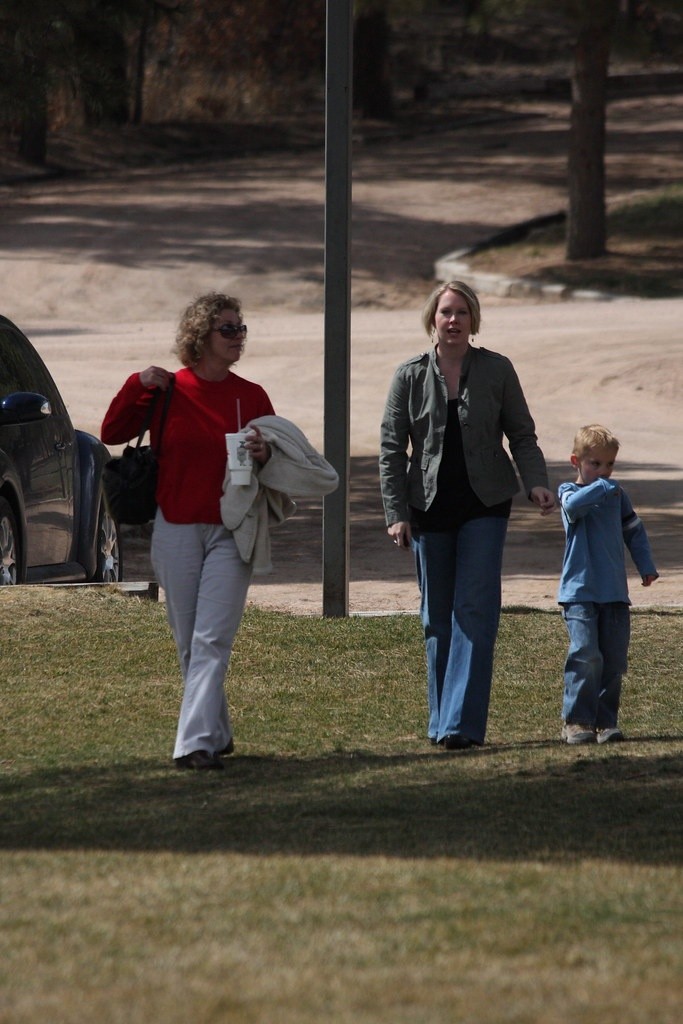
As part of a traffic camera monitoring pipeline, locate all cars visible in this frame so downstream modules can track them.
[0,311,125,588]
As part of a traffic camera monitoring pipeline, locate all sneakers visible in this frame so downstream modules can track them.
[596,725,624,744]
[561,722,598,745]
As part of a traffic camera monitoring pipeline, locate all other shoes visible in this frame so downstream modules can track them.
[175,750,225,771]
[219,738,234,755]
[430,739,442,745]
[444,734,474,749]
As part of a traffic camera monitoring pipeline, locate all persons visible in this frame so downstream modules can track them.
[558,424,660,746]
[378,280,557,750]
[100,290,276,773]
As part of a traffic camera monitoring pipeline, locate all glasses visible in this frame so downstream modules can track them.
[212,323,247,340]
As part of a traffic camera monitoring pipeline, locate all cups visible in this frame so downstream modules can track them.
[224,433,253,485]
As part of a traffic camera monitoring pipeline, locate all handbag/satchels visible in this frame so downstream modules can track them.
[102,371,175,526]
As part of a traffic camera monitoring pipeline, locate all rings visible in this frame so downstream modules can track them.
[259,442,264,450]
[394,539,397,544]
